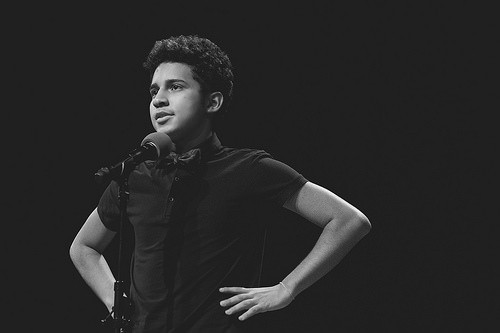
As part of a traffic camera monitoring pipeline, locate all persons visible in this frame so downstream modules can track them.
[69,35,372,333]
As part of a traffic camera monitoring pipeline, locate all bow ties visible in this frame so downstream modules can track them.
[155,148,203,174]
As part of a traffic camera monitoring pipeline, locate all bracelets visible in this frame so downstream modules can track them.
[280,281,295,300]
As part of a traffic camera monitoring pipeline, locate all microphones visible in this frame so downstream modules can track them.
[94,132,173,184]
